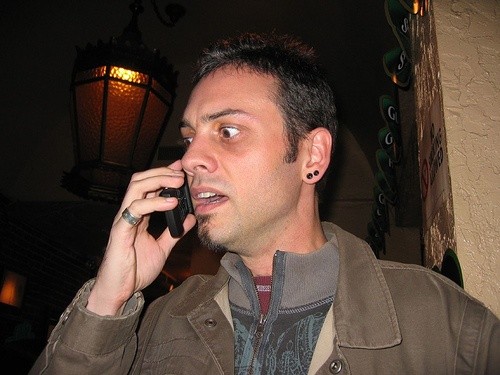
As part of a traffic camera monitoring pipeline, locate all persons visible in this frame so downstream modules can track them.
[31,31,500,374]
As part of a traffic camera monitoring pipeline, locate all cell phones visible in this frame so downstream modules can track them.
[159,167,193,238]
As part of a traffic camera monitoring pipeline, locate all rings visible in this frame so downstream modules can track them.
[120,209,141,228]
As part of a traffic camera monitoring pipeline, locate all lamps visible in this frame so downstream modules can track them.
[61,6,180,206]
